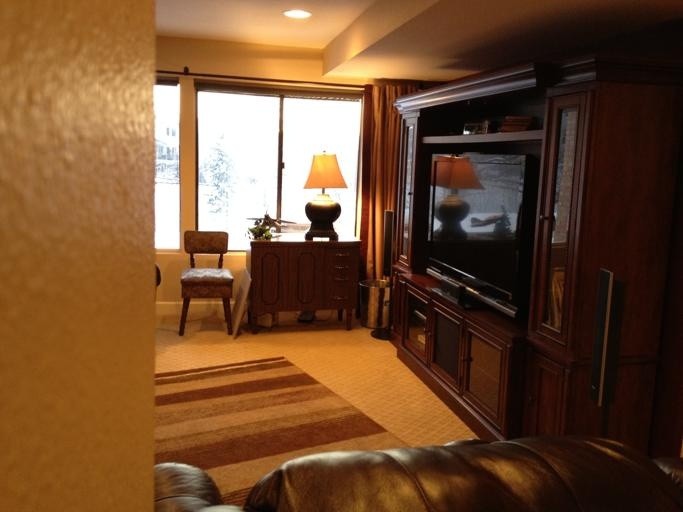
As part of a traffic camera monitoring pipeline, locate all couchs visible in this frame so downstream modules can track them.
[154,434,683,512]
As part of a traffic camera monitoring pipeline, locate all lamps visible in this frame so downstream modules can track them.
[304,151,347,241]
[431,154,485,242]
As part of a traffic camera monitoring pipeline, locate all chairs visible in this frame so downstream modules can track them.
[179,231,234,336]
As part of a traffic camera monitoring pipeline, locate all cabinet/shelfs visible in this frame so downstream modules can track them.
[522,348,657,459]
[391,271,528,442]
[524,81,680,362]
[245,232,362,331]
[394,110,461,270]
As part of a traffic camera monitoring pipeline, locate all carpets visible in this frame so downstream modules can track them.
[155,355,413,510]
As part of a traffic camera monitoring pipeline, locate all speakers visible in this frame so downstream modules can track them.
[589,267,614,408]
[383,210,394,278]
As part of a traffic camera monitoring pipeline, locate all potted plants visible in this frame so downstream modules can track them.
[248,219,272,240]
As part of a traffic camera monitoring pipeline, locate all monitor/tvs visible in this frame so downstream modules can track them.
[424,155,540,317]
